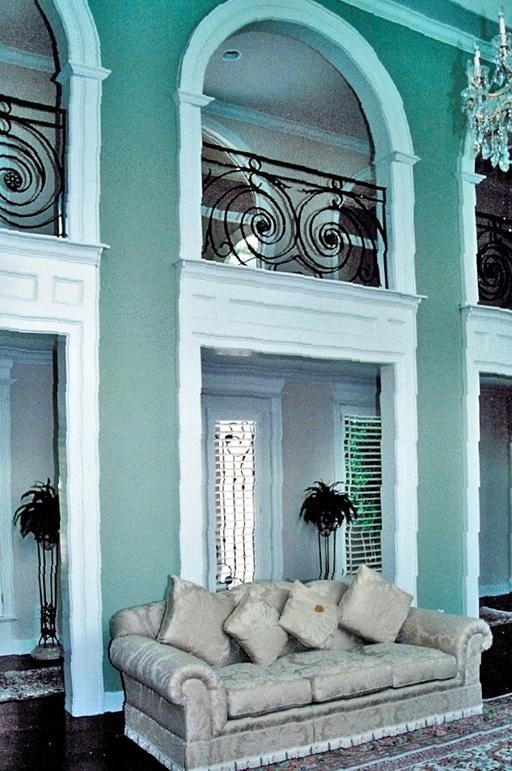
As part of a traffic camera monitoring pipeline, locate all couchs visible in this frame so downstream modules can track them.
[108,581,496,770]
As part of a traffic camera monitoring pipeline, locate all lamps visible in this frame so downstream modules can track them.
[460,5,511,173]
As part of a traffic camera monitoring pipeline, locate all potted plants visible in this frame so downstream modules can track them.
[14,478,61,661]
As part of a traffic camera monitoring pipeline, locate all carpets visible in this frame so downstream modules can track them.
[0,666,65,703]
[247,691,512,770]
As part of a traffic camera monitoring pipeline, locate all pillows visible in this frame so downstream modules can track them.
[338,565,415,644]
[280,580,343,652]
[157,574,234,667]
[221,586,289,665]
